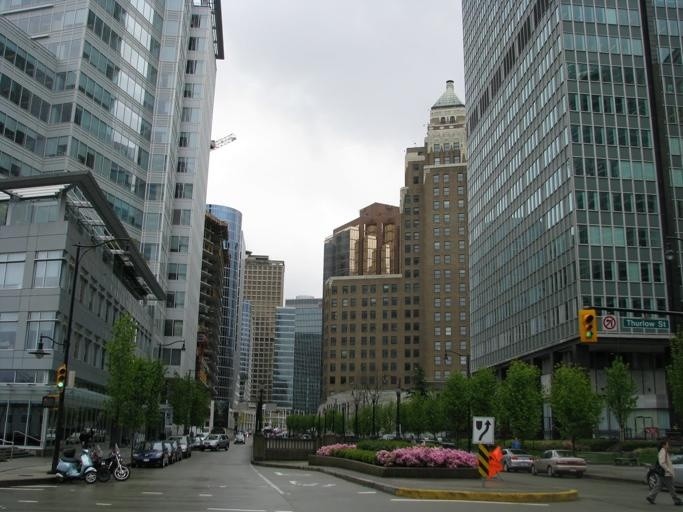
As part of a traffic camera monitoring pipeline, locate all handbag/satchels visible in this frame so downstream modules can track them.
[653,460,666,476]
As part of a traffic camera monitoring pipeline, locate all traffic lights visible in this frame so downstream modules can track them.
[56,366,66,391]
[578,307,598,344]
[42,396,55,409]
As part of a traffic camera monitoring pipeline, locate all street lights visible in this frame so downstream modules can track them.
[442,349,472,451]
[157,340,186,359]
[255,387,266,432]
[370,394,378,439]
[393,386,403,441]
[354,400,361,440]
[24,237,133,477]
[318,403,347,440]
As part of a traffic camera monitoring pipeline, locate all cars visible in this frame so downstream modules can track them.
[501,448,535,474]
[46,423,106,447]
[646,454,682,494]
[132,430,252,469]
[530,449,587,481]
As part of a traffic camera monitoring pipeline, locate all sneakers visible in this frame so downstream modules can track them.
[674,501,683,506]
[646,496,657,504]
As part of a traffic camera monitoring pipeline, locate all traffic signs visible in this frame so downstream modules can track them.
[623,317,669,329]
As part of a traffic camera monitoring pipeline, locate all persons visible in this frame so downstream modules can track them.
[512,437,522,449]
[79,429,88,448]
[645,440,683,505]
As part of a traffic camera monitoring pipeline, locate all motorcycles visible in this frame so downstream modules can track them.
[53,443,130,485]
